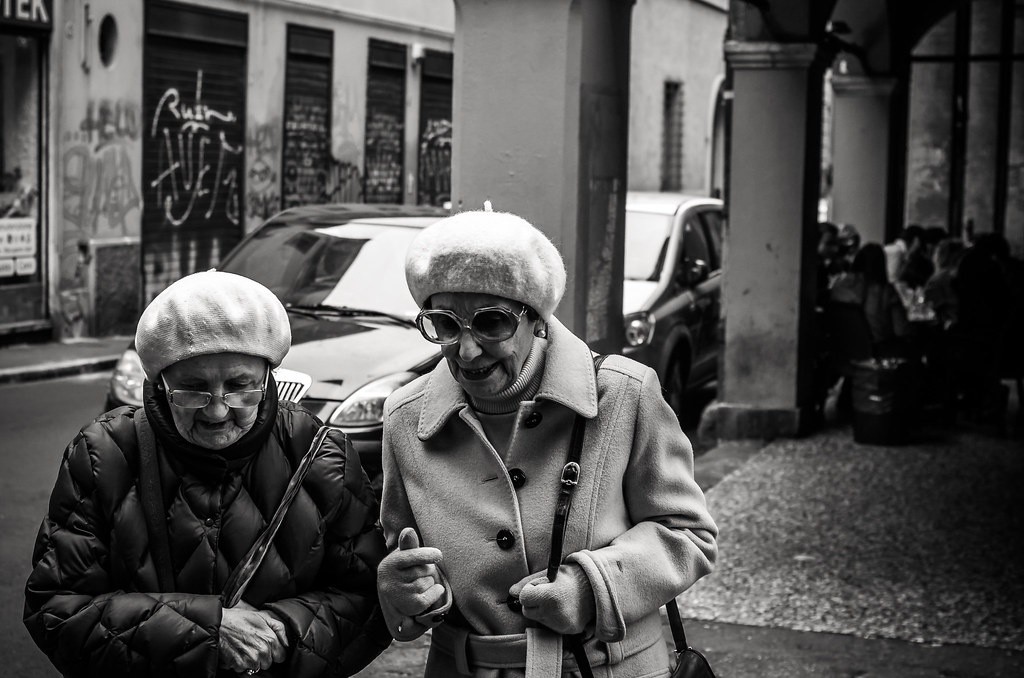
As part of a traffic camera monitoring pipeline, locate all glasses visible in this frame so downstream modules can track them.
[160,365,270,409]
[415,303,528,345]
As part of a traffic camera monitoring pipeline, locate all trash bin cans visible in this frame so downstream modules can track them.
[849,355,919,448]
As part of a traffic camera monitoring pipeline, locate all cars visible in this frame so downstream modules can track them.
[622,191,726,428]
[100,199,453,515]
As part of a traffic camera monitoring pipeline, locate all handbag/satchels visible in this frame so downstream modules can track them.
[667,647,716,678]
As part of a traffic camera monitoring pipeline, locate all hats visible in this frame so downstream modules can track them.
[134,268,292,381]
[404,201,566,324]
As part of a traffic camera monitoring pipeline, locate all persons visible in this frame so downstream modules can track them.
[377,201,720,678]
[23,268,394,678]
[813,223,1024,423]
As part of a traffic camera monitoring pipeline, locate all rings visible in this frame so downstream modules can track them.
[247,667,260,676]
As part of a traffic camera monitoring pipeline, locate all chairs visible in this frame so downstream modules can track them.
[323,239,394,283]
[820,298,1014,423]
